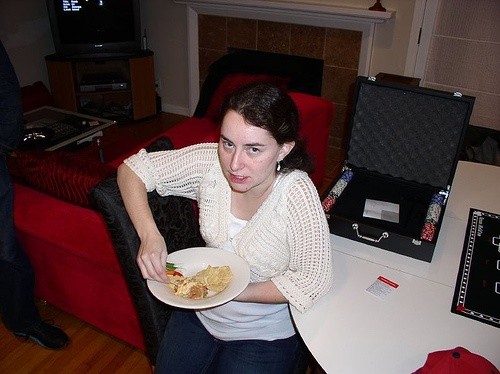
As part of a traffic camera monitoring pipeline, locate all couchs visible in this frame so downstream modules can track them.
[7,74,337,351]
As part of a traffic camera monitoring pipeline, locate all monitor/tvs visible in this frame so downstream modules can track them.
[47,0,141,59]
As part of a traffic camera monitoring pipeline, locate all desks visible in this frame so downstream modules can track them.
[287,161,500,374]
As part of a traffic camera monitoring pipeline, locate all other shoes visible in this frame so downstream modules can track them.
[14,319,71,350]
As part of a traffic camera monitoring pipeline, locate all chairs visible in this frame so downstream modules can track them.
[91,137,310,374]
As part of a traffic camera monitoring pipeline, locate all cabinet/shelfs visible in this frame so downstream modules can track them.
[44,49,159,125]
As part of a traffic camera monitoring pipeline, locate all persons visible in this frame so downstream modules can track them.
[117,81,331,374]
[0,40,71,350]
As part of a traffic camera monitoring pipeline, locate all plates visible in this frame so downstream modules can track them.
[146,246,251,310]
[20,128,56,146]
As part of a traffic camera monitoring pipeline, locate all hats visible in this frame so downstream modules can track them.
[412,346,499,374]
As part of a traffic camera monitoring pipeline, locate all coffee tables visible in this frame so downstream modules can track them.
[20,103,118,164]
